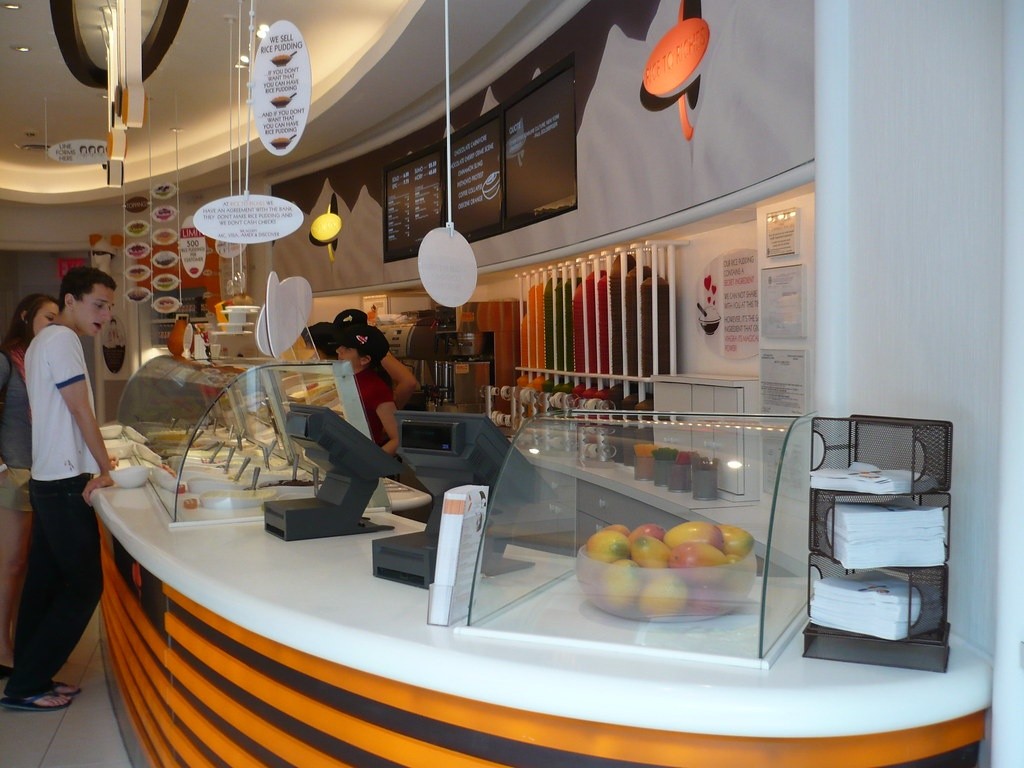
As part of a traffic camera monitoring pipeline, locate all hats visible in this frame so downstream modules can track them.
[333,324,390,361]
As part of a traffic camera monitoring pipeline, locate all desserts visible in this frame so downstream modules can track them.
[128,184,178,310]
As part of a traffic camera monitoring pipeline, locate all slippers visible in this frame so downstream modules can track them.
[50,681,81,695]
[2,693,71,711]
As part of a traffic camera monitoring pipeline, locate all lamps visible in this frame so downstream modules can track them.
[234,20,271,73]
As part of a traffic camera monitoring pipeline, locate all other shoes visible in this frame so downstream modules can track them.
[0,665,14,679]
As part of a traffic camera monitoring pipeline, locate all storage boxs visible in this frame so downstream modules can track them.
[800,413,955,675]
[423,580,472,629]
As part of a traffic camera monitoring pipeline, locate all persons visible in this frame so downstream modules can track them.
[0,266,118,711]
[0,293,61,675]
[301,309,416,479]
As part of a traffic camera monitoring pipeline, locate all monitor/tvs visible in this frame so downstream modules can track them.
[393,408,556,503]
[286,403,406,477]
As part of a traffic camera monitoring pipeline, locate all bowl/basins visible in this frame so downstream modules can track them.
[99,425,322,512]
[269,140,292,149]
[576,545,757,623]
[270,56,292,67]
[270,98,292,109]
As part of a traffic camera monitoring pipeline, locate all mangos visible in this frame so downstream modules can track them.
[587,523,754,614]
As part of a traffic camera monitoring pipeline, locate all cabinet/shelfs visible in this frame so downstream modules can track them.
[649,374,760,502]
[525,456,754,565]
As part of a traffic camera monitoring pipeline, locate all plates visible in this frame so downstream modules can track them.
[125,181,180,313]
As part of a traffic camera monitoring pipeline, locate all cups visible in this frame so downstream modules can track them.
[667,464,692,493]
[691,468,718,500]
[633,455,655,482]
[653,460,674,486]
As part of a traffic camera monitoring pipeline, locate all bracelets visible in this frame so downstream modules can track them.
[0,463,8,473]
